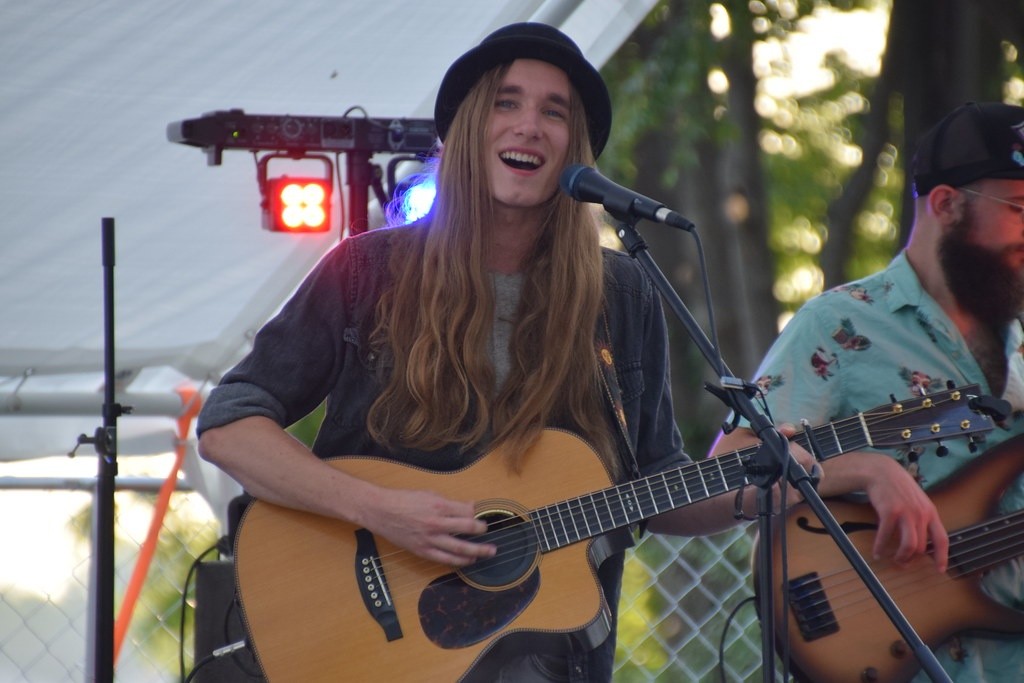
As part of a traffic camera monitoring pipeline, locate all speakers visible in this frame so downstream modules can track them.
[193,560,269,683]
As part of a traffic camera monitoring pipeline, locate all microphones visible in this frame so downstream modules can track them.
[558,162,696,232]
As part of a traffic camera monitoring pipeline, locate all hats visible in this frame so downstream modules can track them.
[435,22,613,164]
[911,102,1024,198]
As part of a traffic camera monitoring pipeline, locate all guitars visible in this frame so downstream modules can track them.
[759,431,1022,683]
[233,375,1011,683]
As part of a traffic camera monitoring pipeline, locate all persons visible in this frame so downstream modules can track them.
[711,95,1024,683]
[198,13,817,683]
